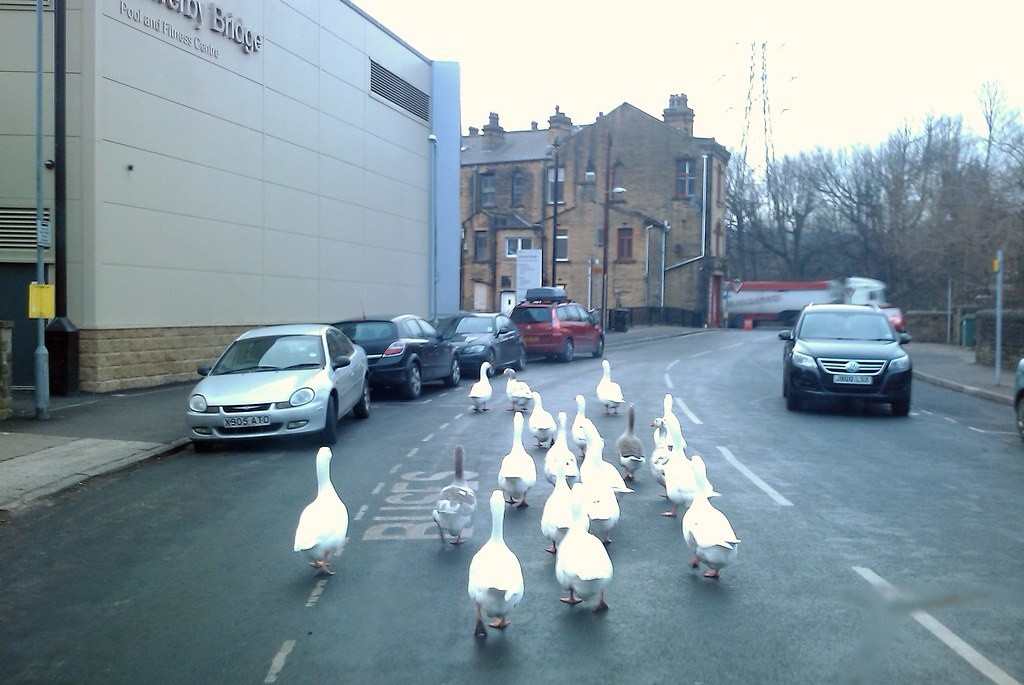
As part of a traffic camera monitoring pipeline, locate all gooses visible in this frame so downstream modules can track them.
[293,445,349,579]
[430,359,742,635]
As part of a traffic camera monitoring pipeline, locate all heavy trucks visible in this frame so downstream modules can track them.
[722,276,888,330]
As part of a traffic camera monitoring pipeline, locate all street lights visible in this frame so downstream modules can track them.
[595,187,627,334]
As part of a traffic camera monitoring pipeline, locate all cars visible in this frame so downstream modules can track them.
[186,323,371,454]
[859,302,911,344]
[778,301,914,417]
[434,311,526,379]
[509,301,605,363]
[1012,357,1024,442]
[330,313,462,401]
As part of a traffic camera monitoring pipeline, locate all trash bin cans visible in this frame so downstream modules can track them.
[615,309,631,331]
